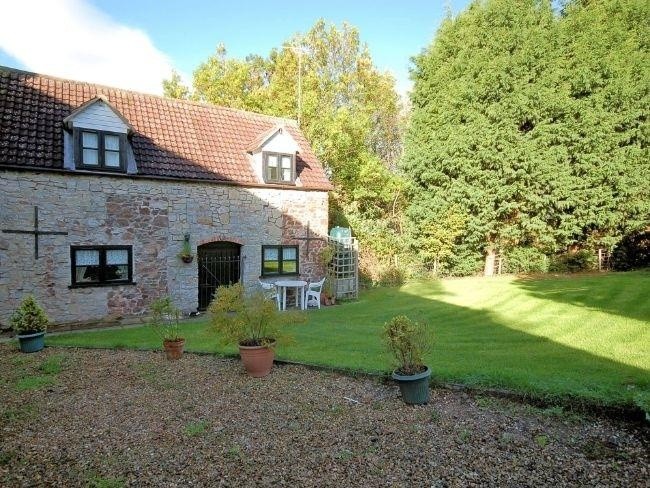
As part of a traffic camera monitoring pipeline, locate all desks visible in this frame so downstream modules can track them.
[275,281,308,311]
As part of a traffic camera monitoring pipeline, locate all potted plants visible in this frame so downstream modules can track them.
[318,245,337,306]
[383,313,434,404]
[181,239,195,264]
[10,296,50,353]
[149,297,186,358]
[209,280,279,377]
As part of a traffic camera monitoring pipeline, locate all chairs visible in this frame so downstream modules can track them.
[304,276,325,310]
